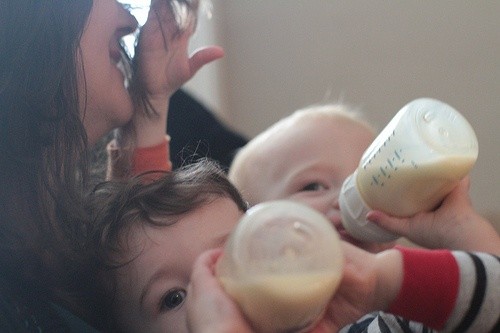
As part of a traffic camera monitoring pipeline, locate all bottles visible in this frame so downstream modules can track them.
[340,99,478,244]
[217,201,342,331]
